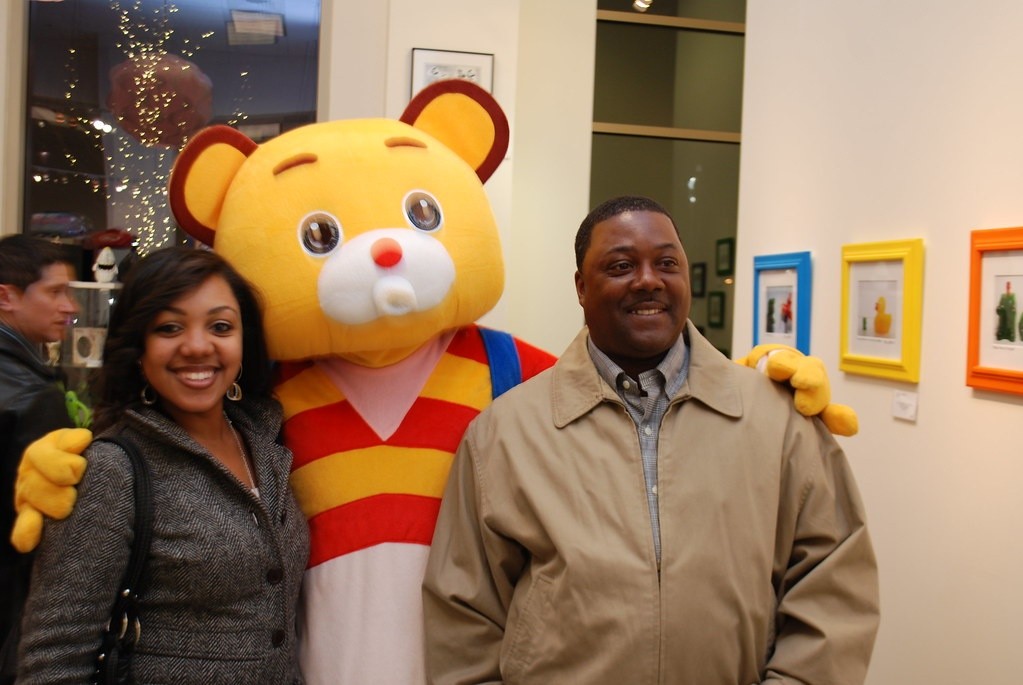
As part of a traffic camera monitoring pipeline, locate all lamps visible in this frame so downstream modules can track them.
[632,0,653,13]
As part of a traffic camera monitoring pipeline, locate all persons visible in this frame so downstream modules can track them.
[13,243,309,685]
[421,193,882,684]
[0,236,83,685]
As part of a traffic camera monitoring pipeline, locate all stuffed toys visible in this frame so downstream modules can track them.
[12,78,861,685]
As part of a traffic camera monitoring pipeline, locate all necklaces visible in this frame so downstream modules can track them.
[226,409,258,497]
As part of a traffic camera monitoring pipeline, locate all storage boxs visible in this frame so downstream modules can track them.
[73,328,108,366]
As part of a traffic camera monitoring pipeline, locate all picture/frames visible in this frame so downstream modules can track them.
[691,263,708,295]
[965,227,1023,397]
[754,251,812,353]
[713,238,735,275]
[838,240,925,383]
[709,291,725,327]
[409,46,495,104]
